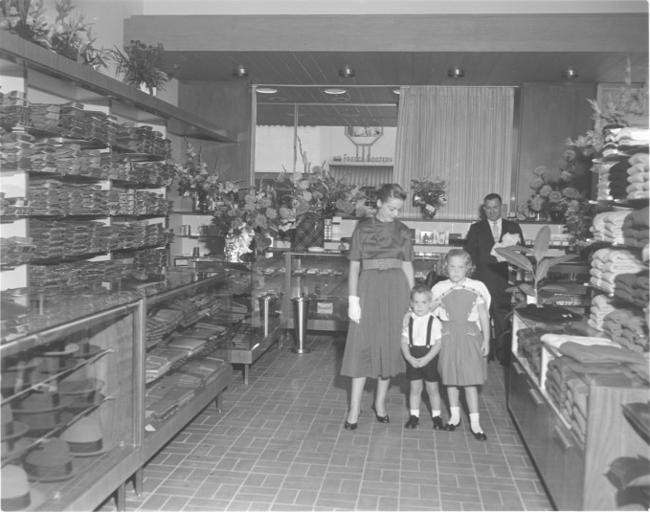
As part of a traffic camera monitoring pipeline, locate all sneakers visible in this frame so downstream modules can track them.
[406,415,419,428]
[432,416,442,430]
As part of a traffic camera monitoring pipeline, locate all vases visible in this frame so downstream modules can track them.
[422,205,436,220]
[292,213,324,253]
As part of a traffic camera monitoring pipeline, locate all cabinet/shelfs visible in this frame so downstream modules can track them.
[505,305,650,512]
[0,29,288,511]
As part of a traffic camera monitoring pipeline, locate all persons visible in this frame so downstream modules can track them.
[430,249,492,440]
[338,182,415,431]
[462,192,527,366]
[400,284,444,430]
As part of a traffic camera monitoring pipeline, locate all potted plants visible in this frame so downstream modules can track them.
[0,0,97,63]
[586,54,649,133]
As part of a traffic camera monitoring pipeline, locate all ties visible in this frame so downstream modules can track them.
[492,222,499,240]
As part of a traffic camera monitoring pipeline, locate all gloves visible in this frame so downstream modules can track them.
[347,295,361,323]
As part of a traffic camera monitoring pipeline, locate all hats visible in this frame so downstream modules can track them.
[1,464,46,512]
[0,332,104,441]
[23,438,76,483]
[59,417,117,457]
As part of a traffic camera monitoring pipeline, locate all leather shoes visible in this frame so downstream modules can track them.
[344,421,357,430]
[444,418,462,431]
[470,425,486,440]
[372,402,389,424]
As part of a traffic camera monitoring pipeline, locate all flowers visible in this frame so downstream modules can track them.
[273,134,372,232]
[515,129,605,242]
[409,175,450,217]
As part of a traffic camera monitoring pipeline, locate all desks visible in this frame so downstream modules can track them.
[282,251,440,355]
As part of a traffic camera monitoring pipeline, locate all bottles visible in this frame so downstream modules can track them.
[180,224,191,237]
[193,246,200,258]
